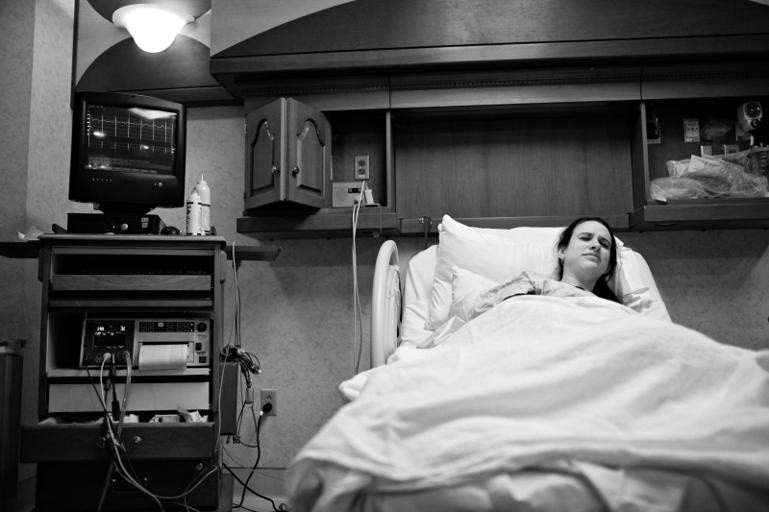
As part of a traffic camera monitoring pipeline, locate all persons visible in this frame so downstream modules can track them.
[555,217,617,292]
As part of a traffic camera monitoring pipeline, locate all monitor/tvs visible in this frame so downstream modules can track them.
[68,91,187,217]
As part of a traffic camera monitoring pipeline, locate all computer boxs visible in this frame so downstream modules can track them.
[65,212,166,236]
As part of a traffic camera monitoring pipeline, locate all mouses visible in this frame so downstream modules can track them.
[160,226,181,236]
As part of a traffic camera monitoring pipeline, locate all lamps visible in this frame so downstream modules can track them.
[88,0,211,53]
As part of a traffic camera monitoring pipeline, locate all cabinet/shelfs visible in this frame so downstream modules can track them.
[33,234,228,512]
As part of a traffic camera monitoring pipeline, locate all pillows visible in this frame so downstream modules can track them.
[424,215,623,328]
[261,389,278,416]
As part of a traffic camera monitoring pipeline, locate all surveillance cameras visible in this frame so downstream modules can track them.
[737,102,763,132]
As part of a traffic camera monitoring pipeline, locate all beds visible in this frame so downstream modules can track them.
[348,215,769,512]
